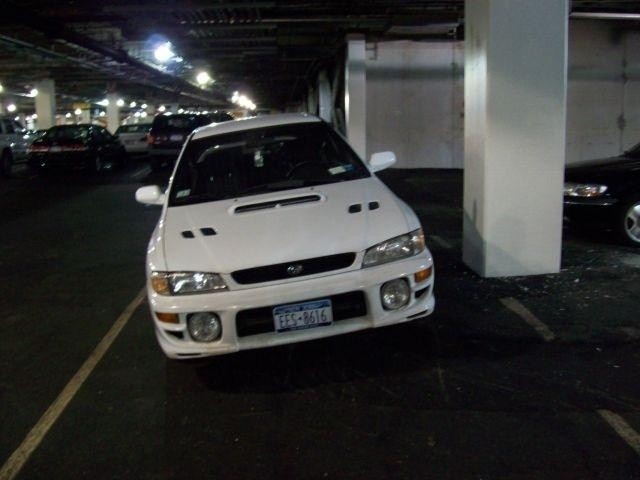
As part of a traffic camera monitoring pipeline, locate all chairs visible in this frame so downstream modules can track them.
[190,156,247,197]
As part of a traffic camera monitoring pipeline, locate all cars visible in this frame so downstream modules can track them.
[564,141,640,251]
[133,114,436,360]
[1,111,235,179]
